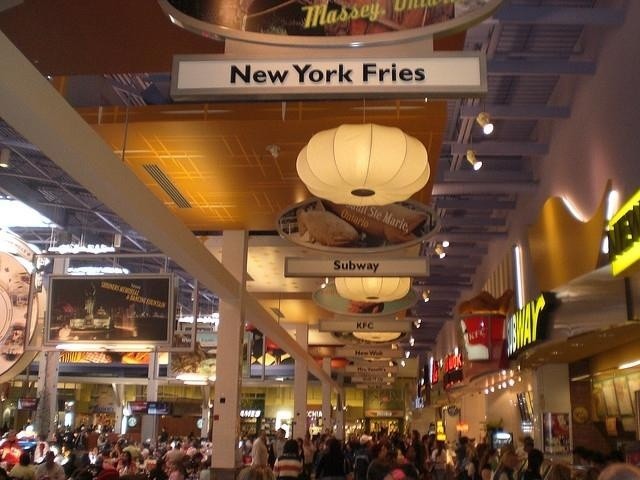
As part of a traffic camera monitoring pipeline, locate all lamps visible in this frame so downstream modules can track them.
[466,150,484,171]
[473,114,494,134]
[297,98,430,206]
[335,277,410,303]
[436,246,446,258]
[423,292,430,303]
